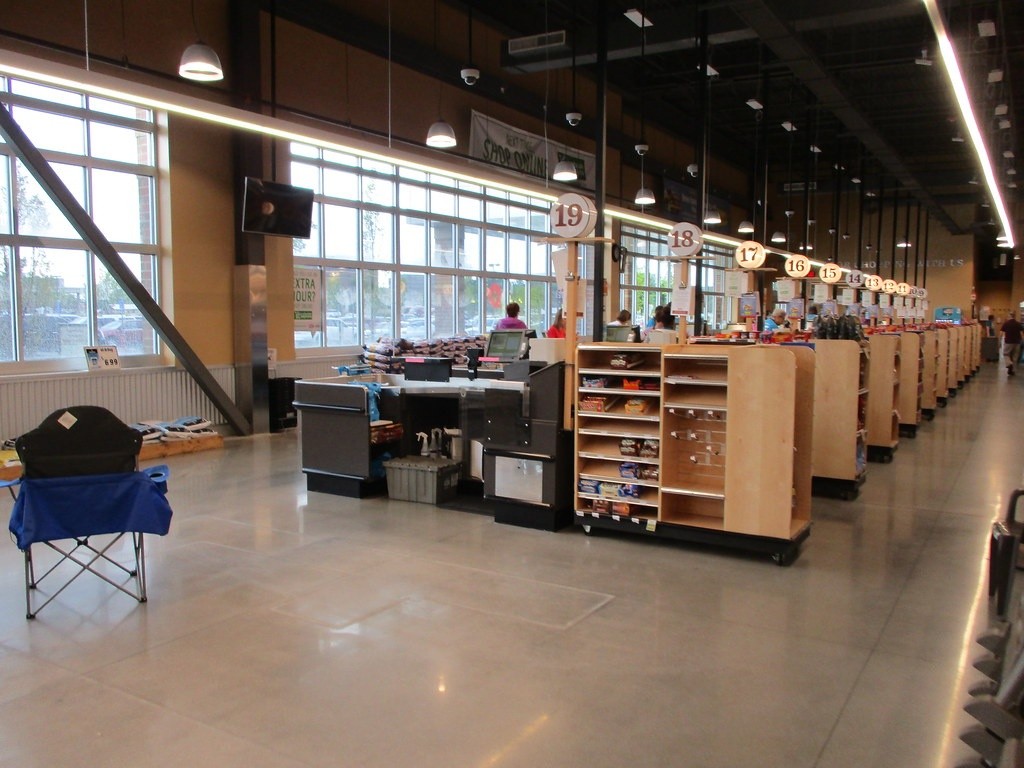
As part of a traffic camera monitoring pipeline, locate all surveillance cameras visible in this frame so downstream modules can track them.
[567,112,582,126]
[635,145,649,155]
[460,69,480,85]
[687,164,698,177]
[785,210,873,250]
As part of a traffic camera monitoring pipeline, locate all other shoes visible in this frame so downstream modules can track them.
[1006,364,1017,376]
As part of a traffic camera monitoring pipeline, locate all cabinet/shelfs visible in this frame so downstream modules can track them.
[573,345,815,561]
[813,322,983,501]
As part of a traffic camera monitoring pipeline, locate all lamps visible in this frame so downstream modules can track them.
[738,217,755,233]
[176,0,226,84]
[551,69,577,186]
[799,241,813,251]
[895,235,913,248]
[996,229,1009,265]
[771,231,787,243]
[425,0,458,148]
[700,208,721,225]
[634,186,656,206]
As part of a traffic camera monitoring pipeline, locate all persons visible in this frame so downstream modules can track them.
[608,310,631,326]
[807,306,818,320]
[644,302,678,343]
[496,303,526,328]
[1000,312,1024,375]
[547,309,578,341]
[764,309,786,331]
[987,315,995,336]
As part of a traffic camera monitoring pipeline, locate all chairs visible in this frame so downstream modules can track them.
[0,406,173,619]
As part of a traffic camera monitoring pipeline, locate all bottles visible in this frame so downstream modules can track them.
[610,354,645,370]
[440,444,449,459]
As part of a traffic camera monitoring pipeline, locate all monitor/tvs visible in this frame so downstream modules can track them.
[606,326,631,342]
[484,329,527,363]
[242,177,314,239]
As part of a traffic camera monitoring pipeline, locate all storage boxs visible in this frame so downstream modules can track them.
[382,458,461,505]
